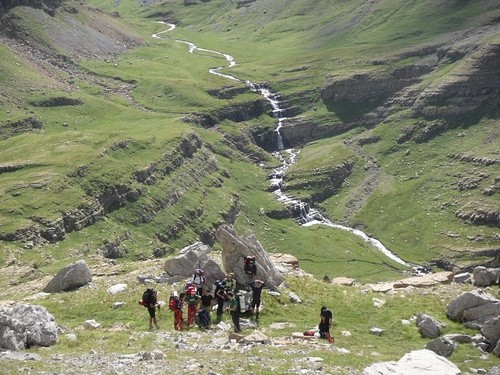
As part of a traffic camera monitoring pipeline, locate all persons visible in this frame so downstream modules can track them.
[244,261,257,286]
[226,287,243,333]
[174,293,185,331]
[148,291,159,330]
[214,275,236,316]
[192,271,203,297]
[319,306,332,339]
[198,289,214,329]
[250,280,265,324]
[185,290,201,326]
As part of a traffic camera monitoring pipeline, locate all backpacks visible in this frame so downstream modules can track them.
[244,256,257,275]
[303,330,315,336]
[169,296,175,311]
[211,280,221,299]
[142,288,153,307]
